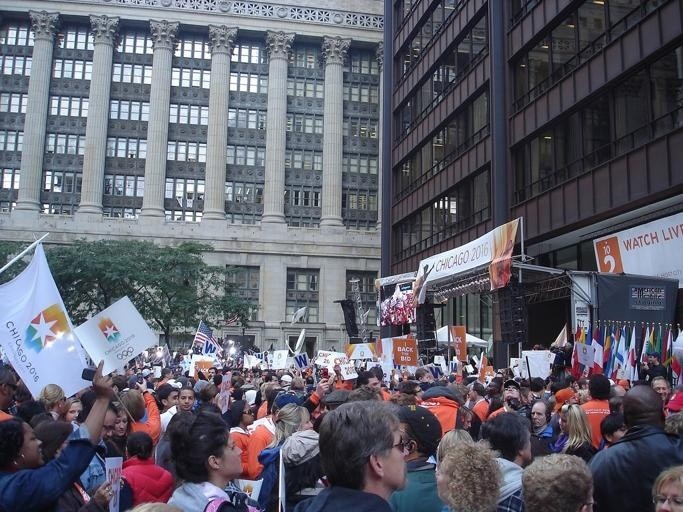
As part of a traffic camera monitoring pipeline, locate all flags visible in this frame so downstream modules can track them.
[71,294,158,377]
[550,326,568,350]
[189,319,222,356]
[372,332,418,365]
[0,244,99,407]
[450,324,467,363]
[224,311,241,328]
[289,306,307,326]
[570,321,681,388]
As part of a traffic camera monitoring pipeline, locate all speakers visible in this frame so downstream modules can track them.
[416,306,436,347]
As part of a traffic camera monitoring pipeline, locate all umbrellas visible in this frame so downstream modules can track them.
[433,324,489,361]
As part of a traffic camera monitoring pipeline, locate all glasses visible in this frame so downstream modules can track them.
[652,495,682,506]
[241,408,253,415]
[505,386,516,391]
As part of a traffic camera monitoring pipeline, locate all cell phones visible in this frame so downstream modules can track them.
[82,368,96,381]
[137,373,143,384]
[320,368,328,383]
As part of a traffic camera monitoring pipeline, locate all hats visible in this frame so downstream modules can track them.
[277,375,291,384]
[323,389,348,404]
[503,380,520,391]
[400,406,442,450]
[141,369,150,377]
[281,430,321,468]
[647,352,659,358]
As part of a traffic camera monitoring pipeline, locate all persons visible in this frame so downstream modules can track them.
[0,341,683,512]
[380,292,415,325]
[495,239,514,287]
[408,264,435,307]
